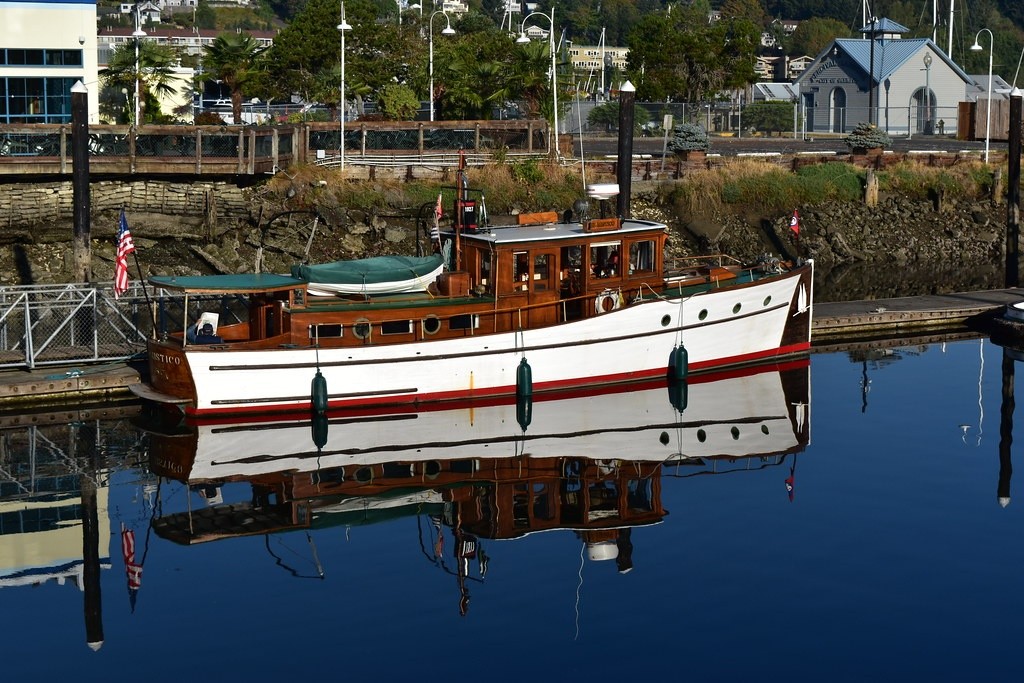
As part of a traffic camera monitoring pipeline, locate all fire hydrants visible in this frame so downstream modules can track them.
[936,119,944,136]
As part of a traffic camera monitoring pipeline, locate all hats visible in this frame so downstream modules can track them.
[202,323,213,336]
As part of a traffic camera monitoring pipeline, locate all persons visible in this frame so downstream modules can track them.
[186,319,225,345]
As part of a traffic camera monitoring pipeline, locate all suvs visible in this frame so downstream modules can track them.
[211,104,272,126]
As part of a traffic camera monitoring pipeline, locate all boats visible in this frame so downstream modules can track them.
[1004,300,1024,320]
[137,356,813,614]
[126,79,815,419]
[291,253,445,296]
[309,488,443,531]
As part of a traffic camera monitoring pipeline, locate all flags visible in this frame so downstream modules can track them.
[433,194,442,221]
[429,513,443,541]
[122,523,143,612]
[434,533,444,556]
[114,207,136,300]
[790,210,799,235]
[785,475,794,502]
[430,210,440,241]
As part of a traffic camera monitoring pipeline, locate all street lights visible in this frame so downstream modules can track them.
[704,104,710,140]
[134,6,148,141]
[398,3,422,31]
[517,13,561,163]
[970,30,993,163]
[429,10,456,122]
[337,0,353,169]
[924,52,932,135]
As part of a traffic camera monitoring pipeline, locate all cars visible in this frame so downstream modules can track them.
[195,99,231,115]
